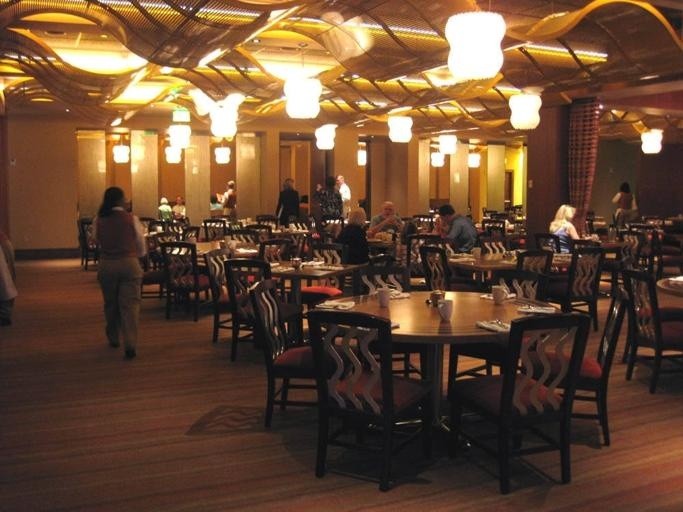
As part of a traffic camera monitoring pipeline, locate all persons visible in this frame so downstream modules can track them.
[159,197,173,223]
[611,182,637,222]
[210,196,222,209]
[92,186,147,358]
[275,175,478,276]
[548,204,594,255]
[173,195,186,220]
[221,180,237,209]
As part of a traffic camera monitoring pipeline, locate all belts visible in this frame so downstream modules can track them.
[101,251,136,259]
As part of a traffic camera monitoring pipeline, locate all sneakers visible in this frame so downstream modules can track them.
[108,339,120,347]
[124,348,136,358]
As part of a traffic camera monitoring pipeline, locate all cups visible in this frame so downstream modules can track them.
[429,288,454,322]
[492,285,509,305]
[376,287,391,308]
[472,247,482,258]
[367,231,375,239]
[218,234,238,251]
[155,226,163,233]
[290,258,302,268]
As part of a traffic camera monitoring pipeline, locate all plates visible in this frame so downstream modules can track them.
[367,239,382,243]
